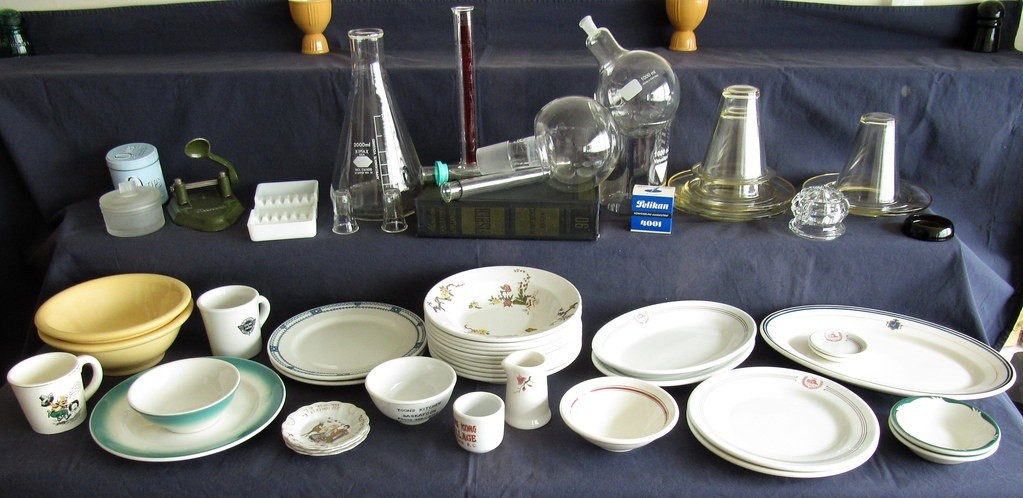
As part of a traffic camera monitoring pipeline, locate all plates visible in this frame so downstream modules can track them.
[282,402,371,457]
[90,357,286,461]
[591,299,757,386]
[423,265,582,384]
[760,303,1016,400]
[687,367,879,478]
[889,396,1002,465]
[267,302,427,386]
[809,331,869,361]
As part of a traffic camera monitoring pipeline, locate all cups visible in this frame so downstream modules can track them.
[332,190,359,235]
[196,285,270,359]
[452,392,504,453]
[381,187,408,232]
[7,351,103,434]
[503,351,552,430]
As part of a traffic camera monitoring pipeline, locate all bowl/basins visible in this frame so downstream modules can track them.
[127,357,241,434]
[364,357,456,425]
[34,273,193,377]
[559,376,679,451]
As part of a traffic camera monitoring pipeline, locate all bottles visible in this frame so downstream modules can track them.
[332,28,425,221]
[579,16,679,133]
[476,96,623,190]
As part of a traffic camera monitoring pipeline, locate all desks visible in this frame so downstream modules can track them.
[0,0,1023,498]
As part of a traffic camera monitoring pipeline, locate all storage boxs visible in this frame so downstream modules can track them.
[630,184,676,234]
[414,176,599,240]
